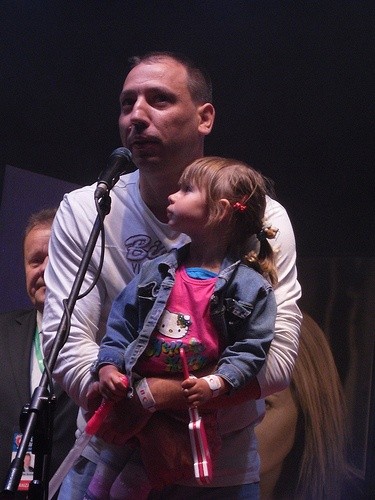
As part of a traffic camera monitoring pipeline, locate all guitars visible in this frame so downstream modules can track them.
[180,347,217,486]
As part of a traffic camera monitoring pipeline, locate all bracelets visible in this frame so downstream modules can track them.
[200,374,222,399]
[134,375,159,415]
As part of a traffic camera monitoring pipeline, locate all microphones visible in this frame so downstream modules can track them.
[93,146,133,199]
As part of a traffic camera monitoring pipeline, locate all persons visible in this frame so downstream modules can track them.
[0,208,81,500]
[41,51,302,500]
[21,453,33,472]
[255,314,364,500]
[85,157,280,500]
